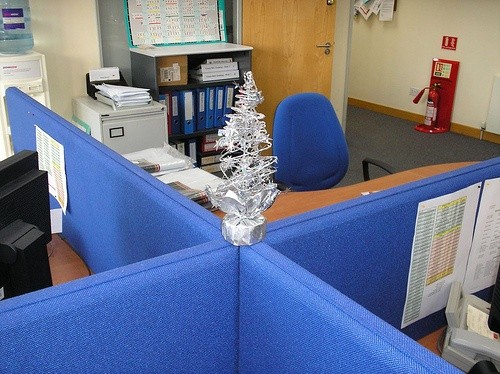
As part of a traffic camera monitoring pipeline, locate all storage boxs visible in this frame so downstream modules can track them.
[156,55,188,87]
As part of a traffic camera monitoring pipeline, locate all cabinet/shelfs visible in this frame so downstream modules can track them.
[129,43,255,177]
[72,94,168,155]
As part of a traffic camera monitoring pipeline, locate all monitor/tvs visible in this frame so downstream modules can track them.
[0,149,54,302]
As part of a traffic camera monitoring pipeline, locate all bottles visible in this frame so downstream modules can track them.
[0,0,34,53]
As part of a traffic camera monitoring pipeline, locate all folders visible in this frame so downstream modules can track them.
[171,85,235,134]
[192,62,240,82]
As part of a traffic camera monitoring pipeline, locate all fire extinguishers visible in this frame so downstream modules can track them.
[423,81,443,129]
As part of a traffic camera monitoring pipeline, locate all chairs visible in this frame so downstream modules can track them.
[271,93,395,191]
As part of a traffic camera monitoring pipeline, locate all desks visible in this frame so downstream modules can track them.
[213,162,478,223]
[46,233,92,286]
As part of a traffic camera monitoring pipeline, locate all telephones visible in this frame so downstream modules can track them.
[441,279,500,373]
